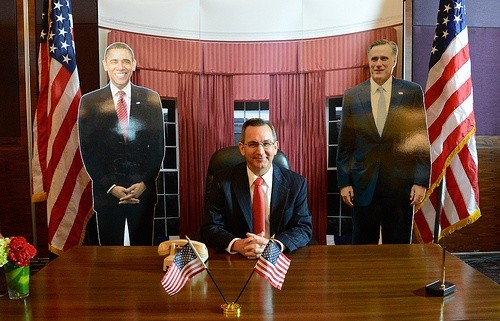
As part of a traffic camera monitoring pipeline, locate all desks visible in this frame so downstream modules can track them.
[0,244,500,321]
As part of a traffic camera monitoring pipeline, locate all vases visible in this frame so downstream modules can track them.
[4,260,30,299]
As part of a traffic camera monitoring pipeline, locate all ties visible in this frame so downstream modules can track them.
[252,177,265,234]
[377,87,388,137]
[116,91,129,142]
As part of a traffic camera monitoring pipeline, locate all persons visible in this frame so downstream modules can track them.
[203,118,313,261]
[336,39,432,246]
[77,42,165,246]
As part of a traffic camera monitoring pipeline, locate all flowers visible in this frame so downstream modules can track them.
[0,235,37,267]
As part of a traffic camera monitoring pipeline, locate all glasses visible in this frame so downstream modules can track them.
[244,142,275,148]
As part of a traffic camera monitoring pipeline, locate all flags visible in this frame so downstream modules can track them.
[160,242,206,296]
[32,1,95,255]
[414,0,482,245]
[254,239,291,290]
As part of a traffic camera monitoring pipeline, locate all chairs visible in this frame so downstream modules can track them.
[199,144,319,245]
